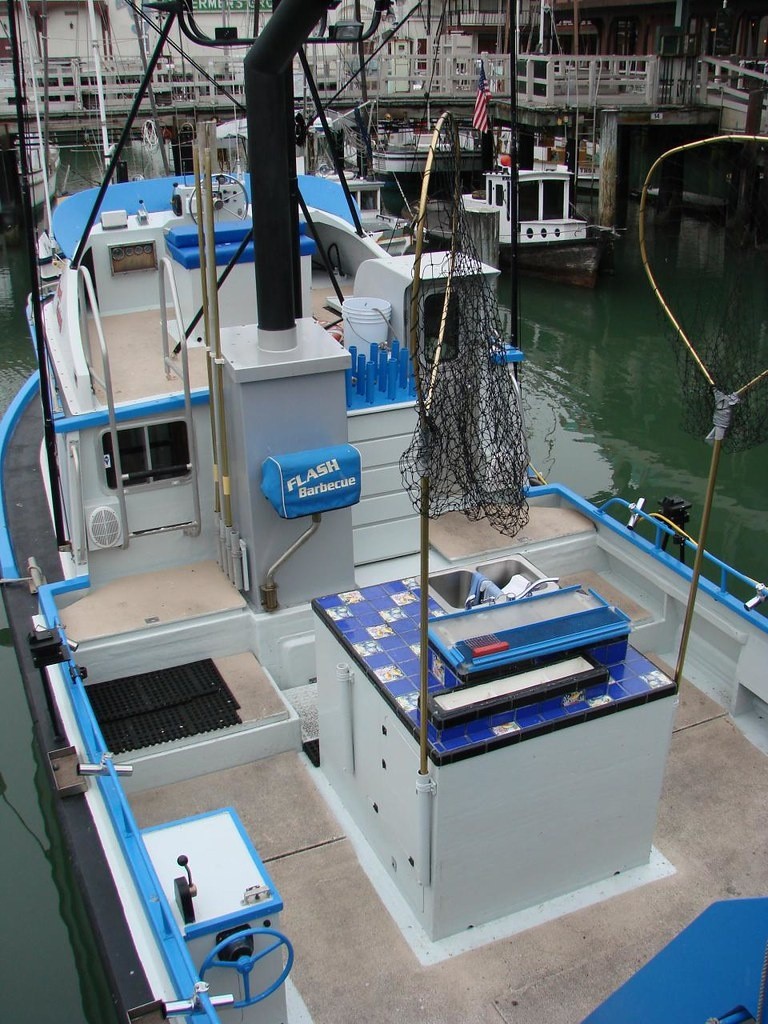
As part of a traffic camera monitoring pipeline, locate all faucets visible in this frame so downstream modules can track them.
[505,577,560,601]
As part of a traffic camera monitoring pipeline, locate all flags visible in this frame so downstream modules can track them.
[471,59,494,135]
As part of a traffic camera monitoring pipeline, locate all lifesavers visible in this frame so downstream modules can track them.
[316,319,344,344]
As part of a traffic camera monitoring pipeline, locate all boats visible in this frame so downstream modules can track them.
[0,0,768,1024]
[461,153,590,245]
[215,106,384,211]
[8,132,61,209]
[342,117,496,176]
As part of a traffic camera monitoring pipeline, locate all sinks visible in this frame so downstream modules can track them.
[476,559,548,597]
[427,566,496,609]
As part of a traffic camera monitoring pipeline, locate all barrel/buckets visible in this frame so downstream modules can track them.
[341,298,393,367]
